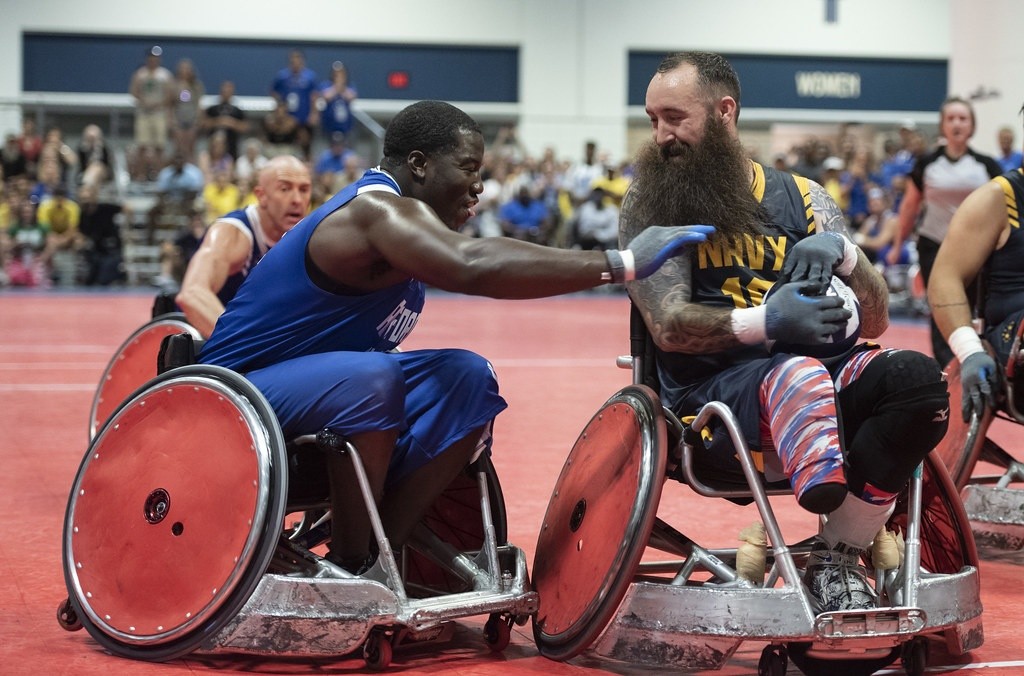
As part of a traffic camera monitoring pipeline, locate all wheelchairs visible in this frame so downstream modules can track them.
[85,291,212,450]
[531,298,987,674]
[930,317,1023,564]
[56,329,542,672]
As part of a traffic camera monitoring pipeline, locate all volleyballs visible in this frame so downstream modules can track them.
[760,270,861,367]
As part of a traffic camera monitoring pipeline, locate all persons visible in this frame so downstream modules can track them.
[616,52,950,616]
[936,156,1024,421]
[190,98,715,579]
[882,97,1007,411]
[173,155,313,343]
[3,34,1024,295]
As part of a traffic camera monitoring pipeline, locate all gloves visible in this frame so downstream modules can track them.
[945,327,1000,418]
[605,223,715,283]
[729,282,851,344]
[781,233,857,292]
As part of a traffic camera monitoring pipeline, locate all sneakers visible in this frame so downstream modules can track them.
[803,537,874,623]
